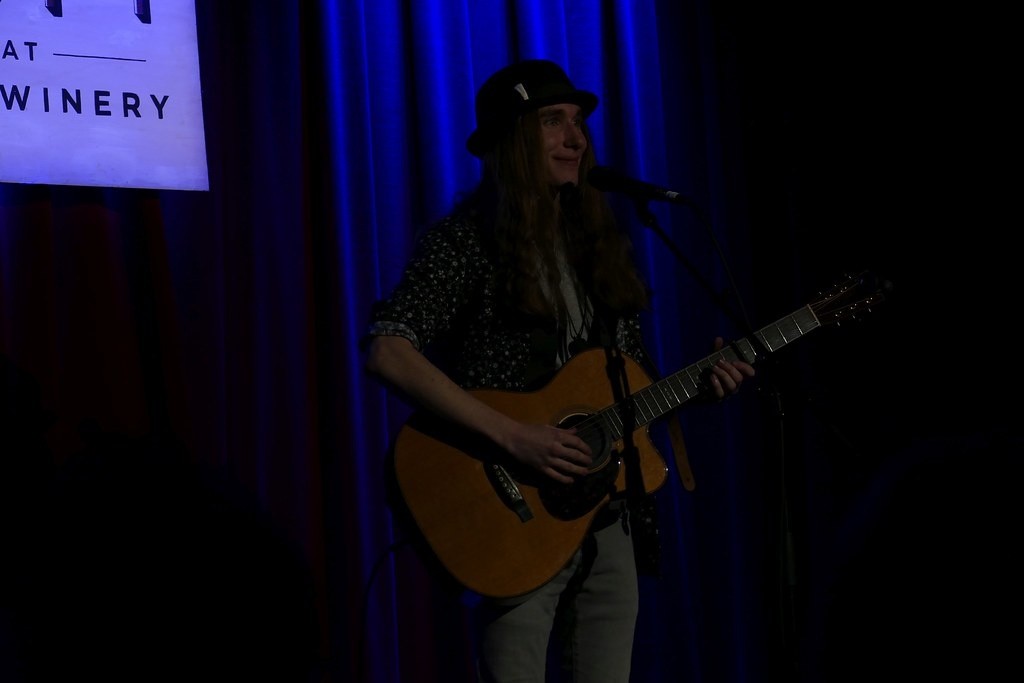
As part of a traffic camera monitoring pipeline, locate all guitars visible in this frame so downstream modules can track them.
[384,261,892,623]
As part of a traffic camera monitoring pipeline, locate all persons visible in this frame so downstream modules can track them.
[359,63,756,682]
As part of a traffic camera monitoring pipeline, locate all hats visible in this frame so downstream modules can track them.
[464,59,600,154]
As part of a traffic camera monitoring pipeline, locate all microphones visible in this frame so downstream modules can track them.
[588,165,680,200]
